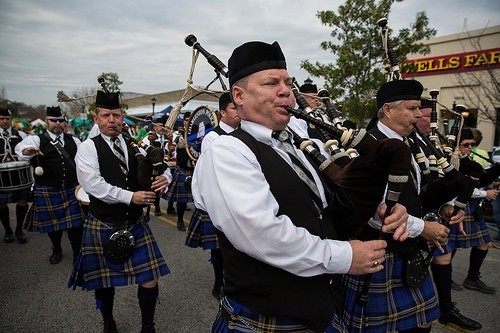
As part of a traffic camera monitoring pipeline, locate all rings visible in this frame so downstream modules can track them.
[373,259,379,267]
[148,199,151,204]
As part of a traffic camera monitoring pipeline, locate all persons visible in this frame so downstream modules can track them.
[0,107,35,245]
[190,42,409,333]
[75,90,173,332]
[0,81,499,333]
[15,105,85,265]
[343,80,467,333]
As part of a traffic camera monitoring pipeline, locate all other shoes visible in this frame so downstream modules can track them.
[49,248,63,265]
[212,286,225,302]
[4,228,27,243]
[177,207,186,231]
[450,276,498,294]
[166,206,176,215]
[438,302,482,331]
[100,319,157,333]
[153,207,162,216]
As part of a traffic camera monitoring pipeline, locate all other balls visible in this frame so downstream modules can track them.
[35,166,43,176]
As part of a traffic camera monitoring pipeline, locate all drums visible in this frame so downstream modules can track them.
[75,184,90,223]
[184,104,223,161]
[0,161,33,192]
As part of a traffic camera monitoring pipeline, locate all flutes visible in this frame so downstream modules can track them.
[376,17,500,268]
[113,90,186,223]
[184,33,412,308]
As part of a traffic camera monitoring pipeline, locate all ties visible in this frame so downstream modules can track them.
[109,136,129,176]
[270,129,323,212]
[2,129,10,139]
[54,136,63,145]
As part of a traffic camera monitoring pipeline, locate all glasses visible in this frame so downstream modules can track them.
[47,118,65,123]
[185,120,189,121]
[460,142,476,148]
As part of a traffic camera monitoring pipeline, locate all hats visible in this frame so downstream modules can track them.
[183,112,192,119]
[96,90,124,110]
[0,108,12,116]
[46,106,64,118]
[376,78,435,110]
[456,128,475,140]
[227,40,288,88]
[153,118,165,124]
[299,83,318,94]
[219,92,232,106]
